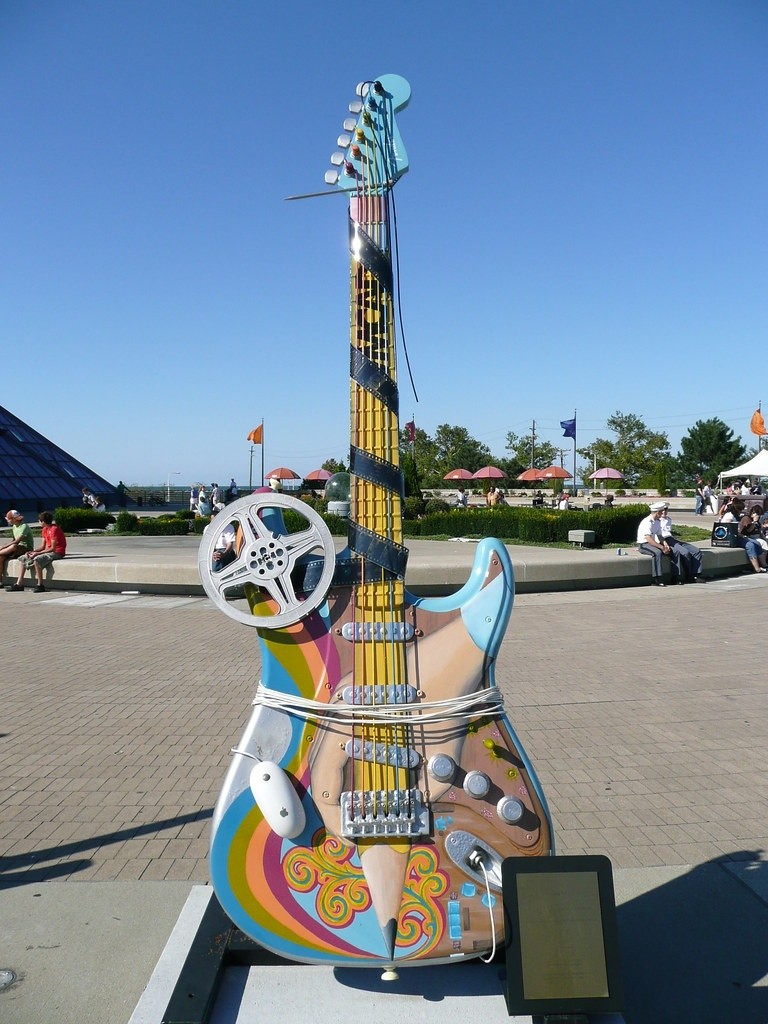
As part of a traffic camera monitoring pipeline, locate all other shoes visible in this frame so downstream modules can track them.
[0,582,4,588]
[651,579,665,586]
[5,584,24,591]
[33,585,45,592]
[755,566,768,573]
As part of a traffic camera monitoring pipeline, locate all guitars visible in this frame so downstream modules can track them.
[199,70,556,983]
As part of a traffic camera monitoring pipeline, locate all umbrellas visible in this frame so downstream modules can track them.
[264,468,301,490]
[516,469,548,499]
[304,470,333,490]
[444,469,477,486]
[471,466,508,486]
[534,466,574,496]
[588,468,625,497]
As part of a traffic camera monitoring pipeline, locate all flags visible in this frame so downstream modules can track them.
[560,408,576,440]
[751,400,768,435]
[247,418,264,444]
[405,412,415,441]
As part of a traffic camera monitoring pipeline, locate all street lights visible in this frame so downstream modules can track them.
[167,470,181,502]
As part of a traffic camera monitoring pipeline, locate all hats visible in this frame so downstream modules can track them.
[663,501,670,508]
[649,502,665,512]
[6,510,24,522]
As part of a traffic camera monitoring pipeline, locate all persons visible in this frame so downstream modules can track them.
[227,478,236,497]
[727,478,762,495]
[190,483,220,517]
[559,494,569,510]
[457,486,467,508]
[695,478,717,517]
[637,501,707,586]
[202,511,236,573]
[0,510,67,592]
[718,496,745,523]
[486,485,503,506]
[738,505,768,573]
[82,487,106,512]
[117,481,131,508]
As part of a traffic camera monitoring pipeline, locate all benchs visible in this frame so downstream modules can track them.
[583,502,622,511]
[518,503,541,509]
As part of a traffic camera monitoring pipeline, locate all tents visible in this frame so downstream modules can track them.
[718,449,768,495]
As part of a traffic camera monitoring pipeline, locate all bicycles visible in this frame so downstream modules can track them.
[149,496,168,507]
